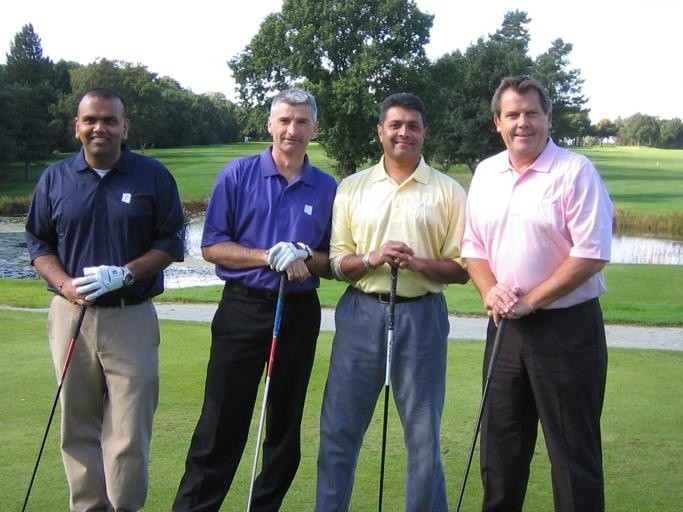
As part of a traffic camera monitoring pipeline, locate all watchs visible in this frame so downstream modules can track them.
[120,265,135,287]
[304,244,312,262]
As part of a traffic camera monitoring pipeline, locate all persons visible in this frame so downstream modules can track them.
[243,134,264,142]
[24,86,186,512]
[312,90,469,512]
[459,75,614,512]
[557,134,617,147]
[140,142,147,154]
[171,88,337,512]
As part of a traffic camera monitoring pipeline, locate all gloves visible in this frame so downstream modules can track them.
[266,241,313,273]
[71,264,124,303]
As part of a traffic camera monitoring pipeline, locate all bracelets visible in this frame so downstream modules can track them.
[361,250,377,272]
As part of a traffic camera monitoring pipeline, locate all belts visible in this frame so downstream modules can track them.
[361,290,432,304]
[91,296,149,307]
[229,284,317,302]
